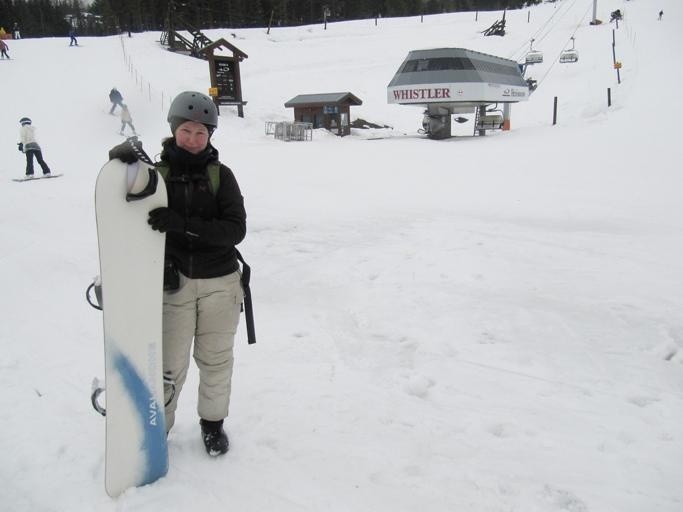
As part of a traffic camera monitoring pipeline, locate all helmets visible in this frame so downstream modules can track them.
[167,91,218,129]
[20,118,31,126]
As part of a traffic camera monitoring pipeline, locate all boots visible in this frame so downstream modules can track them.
[200,418,228,457]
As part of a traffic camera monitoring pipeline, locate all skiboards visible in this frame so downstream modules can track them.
[115,129,142,139]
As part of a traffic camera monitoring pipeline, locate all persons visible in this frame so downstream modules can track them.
[108,92,247,458]
[0,39,10,60]
[658,10,664,20]
[119,104,136,135]
[109,86,123,115]
[68,30,80,46]
[18,117,51,178]
[12,22,22,40]
[609,9,623,29]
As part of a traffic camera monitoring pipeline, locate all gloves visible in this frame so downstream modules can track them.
[148,208,186,234]
[109,136,142,164]
[19,143,23,150]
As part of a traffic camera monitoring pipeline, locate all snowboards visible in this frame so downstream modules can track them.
[11,173,63,182]
[1,58,13,60]
[86,156,168,499]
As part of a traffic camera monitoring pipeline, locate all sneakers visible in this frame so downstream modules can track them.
[27,172,51,178]
